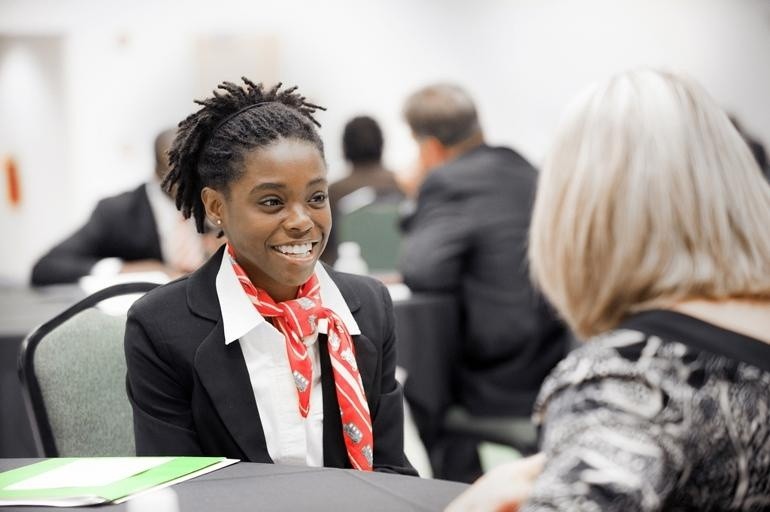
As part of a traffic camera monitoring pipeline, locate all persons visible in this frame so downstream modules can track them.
[458,65,770,512]
[398,82,571,485]
[30,129,227,286]
[125,75,419,476]
[319,116,403,267]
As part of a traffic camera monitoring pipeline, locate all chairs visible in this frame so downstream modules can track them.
[17,280,168,455]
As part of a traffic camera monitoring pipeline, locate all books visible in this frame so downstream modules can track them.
[0,456,240,507]
